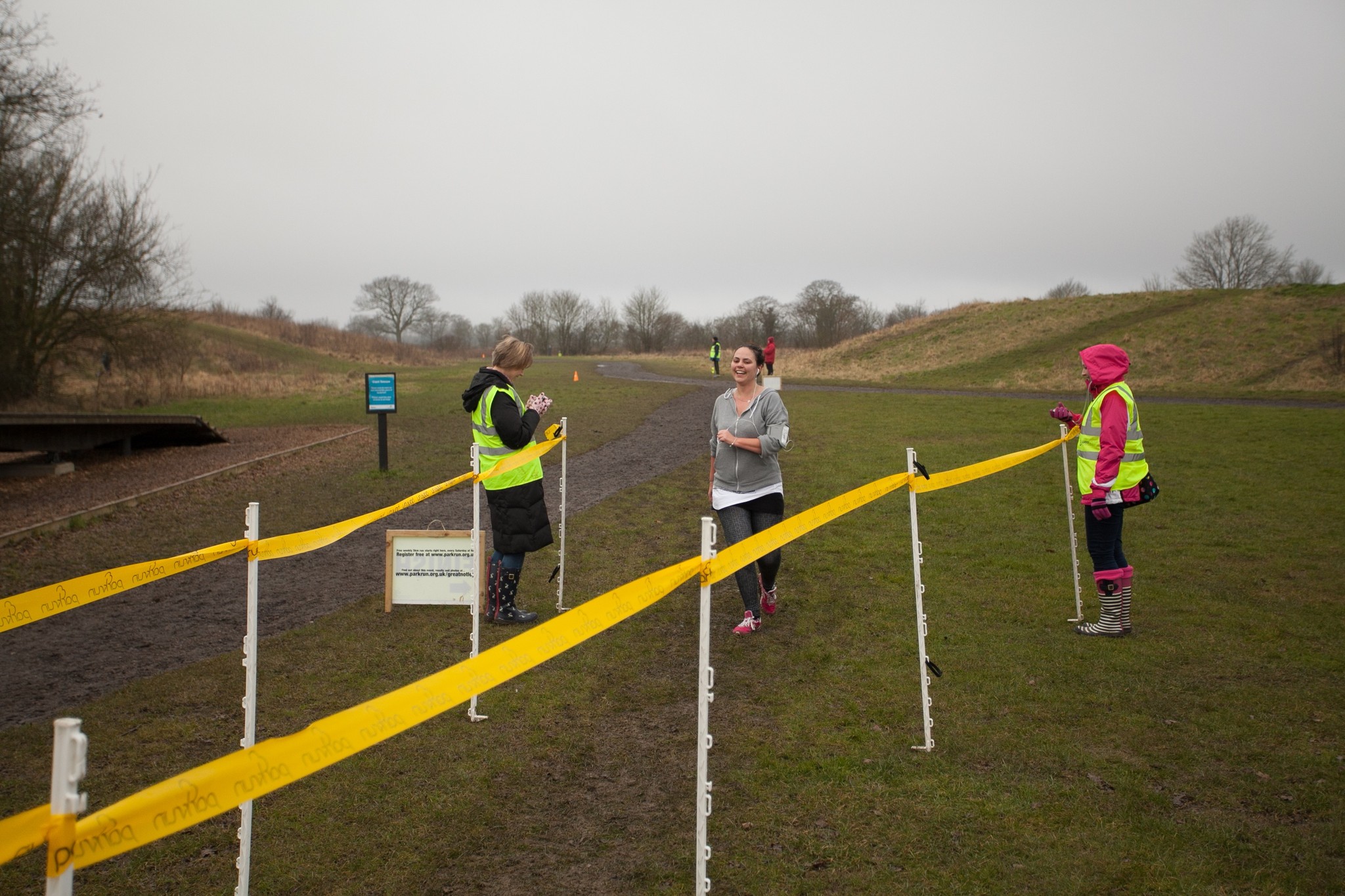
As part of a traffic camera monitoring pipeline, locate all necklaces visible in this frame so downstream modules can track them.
[735,385,758,403]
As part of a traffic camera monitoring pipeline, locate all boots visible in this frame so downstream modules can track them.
[1074,569,1125,638]
[484,556,527,622]
[1119,565,1134,632]
[493,560,538,625]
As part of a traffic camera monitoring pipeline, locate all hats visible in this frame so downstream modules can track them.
[767,337,774,342]
[713,337,718,341]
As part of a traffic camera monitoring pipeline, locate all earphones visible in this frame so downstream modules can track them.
[756,368,760,375]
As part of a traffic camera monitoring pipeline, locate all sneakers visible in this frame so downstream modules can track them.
[731,611,762,636]
[758,573,777,615]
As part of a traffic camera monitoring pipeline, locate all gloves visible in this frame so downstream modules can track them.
[1049,402,1072,422]
[1091,498,1111,520]
[525,392,553,418]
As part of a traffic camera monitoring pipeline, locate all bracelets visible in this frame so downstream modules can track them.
[729,437,738,448]
[709,480,714,484]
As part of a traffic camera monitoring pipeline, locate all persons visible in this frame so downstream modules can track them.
[707,344,789,637]
[710,337,722,378]
[761,337,775,376]
[1049,345,1149,636]
[461,334,553,625]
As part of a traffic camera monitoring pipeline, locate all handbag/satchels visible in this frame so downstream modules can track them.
[1122,471,1160,509]
